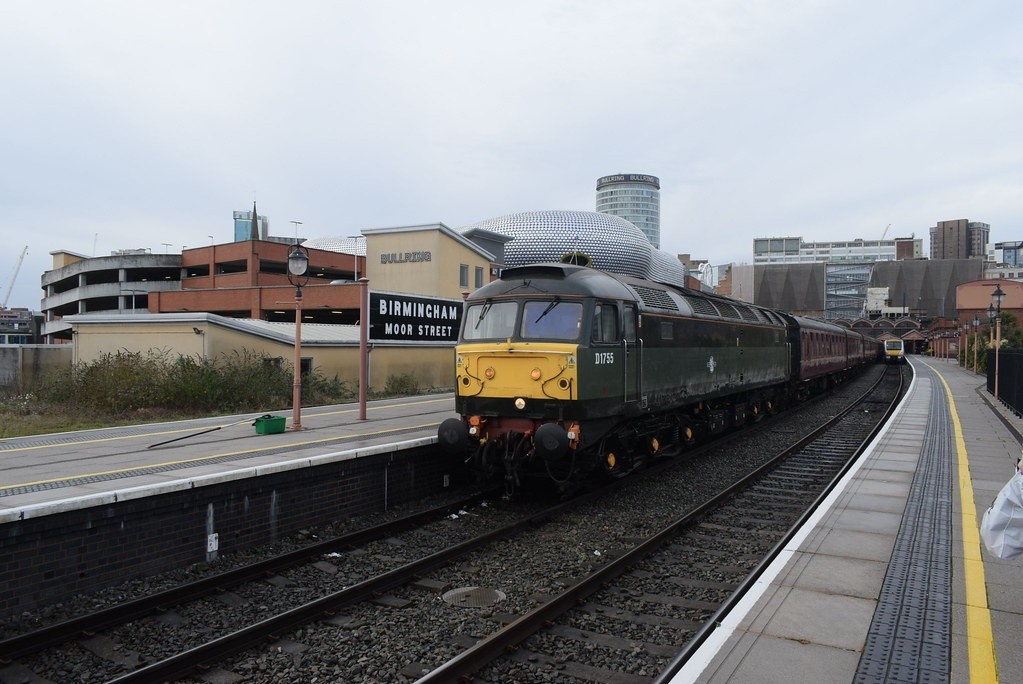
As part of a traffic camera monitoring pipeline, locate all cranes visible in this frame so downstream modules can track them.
[2,245,30,309]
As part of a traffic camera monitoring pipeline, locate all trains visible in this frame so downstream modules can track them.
[438,235,884,503]
[884,339,905,364]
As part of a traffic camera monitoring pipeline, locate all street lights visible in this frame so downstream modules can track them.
[287,239,312,430]
[923,283,1007,406]
